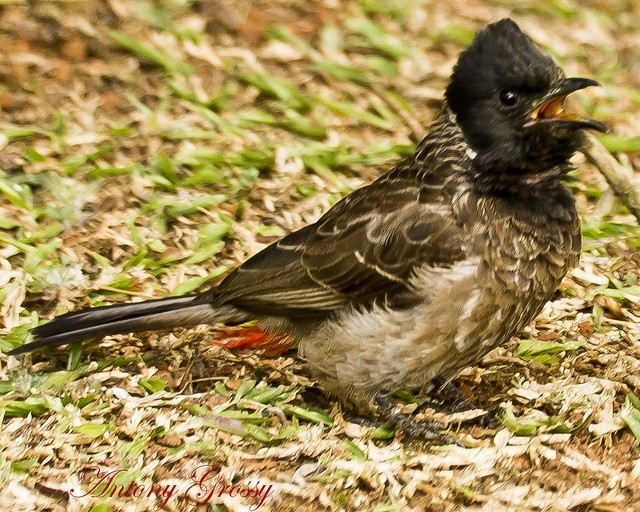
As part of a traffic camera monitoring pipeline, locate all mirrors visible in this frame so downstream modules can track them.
[7,18,611,445]
